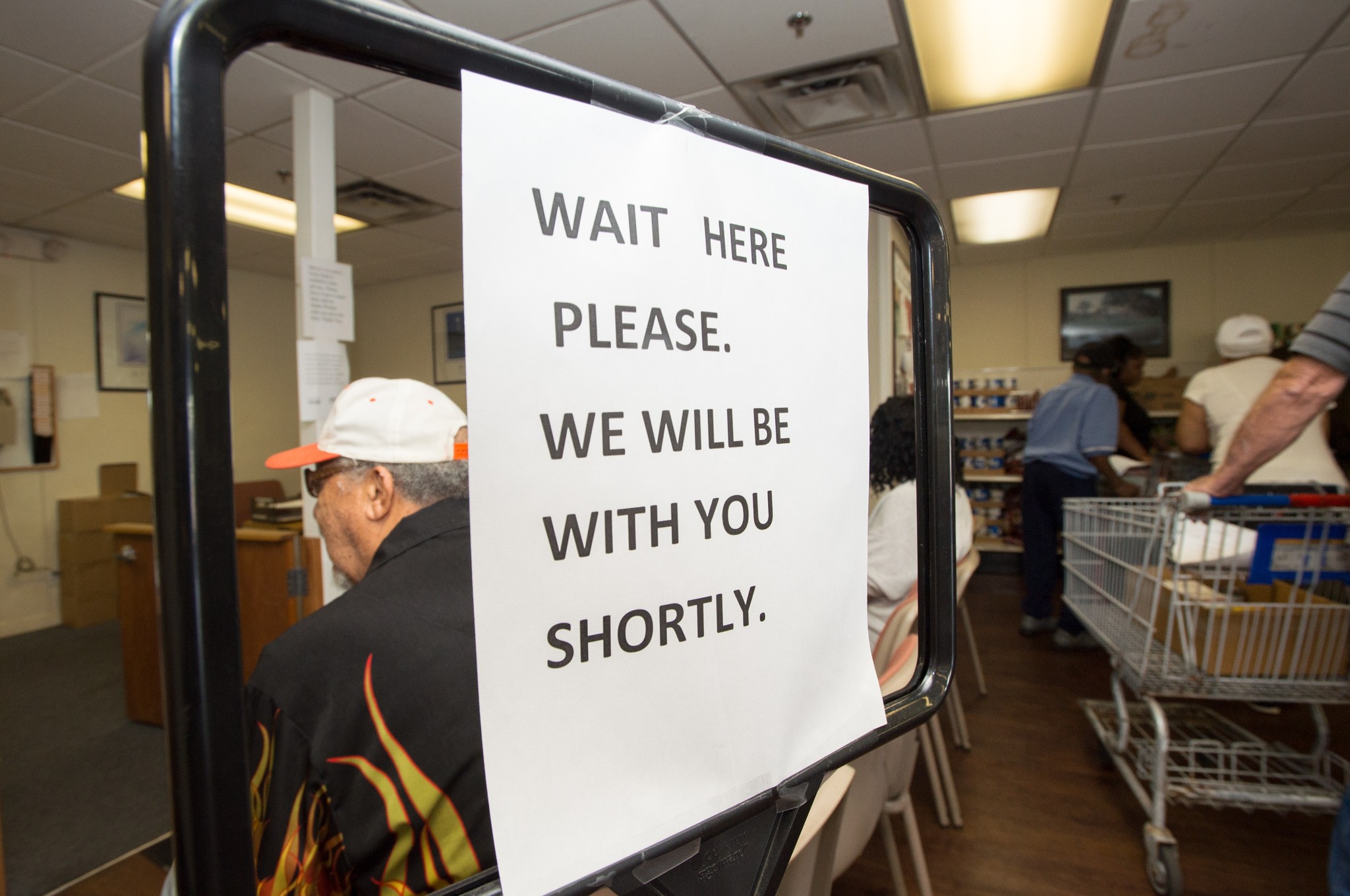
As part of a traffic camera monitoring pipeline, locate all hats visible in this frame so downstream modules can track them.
[1214,314,1274,358]
[264,377,468,469]
[1072,341,1116,370]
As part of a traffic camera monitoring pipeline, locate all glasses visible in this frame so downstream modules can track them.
[304,461,371,498]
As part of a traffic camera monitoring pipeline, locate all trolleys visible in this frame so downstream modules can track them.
[1059,480,1350,896]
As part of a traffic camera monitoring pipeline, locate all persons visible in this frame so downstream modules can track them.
[1021,342,1139,647]
[1177,314,1350,715]
[1098,335,1149,460]
[869,395,971,656]
[1176,267,1350,896]
[159,375,501,896]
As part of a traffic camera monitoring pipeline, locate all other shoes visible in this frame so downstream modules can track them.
[1018,613,1057,634]
[1055,627,1098,647]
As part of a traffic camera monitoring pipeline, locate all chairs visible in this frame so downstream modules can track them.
[786,547,993,896]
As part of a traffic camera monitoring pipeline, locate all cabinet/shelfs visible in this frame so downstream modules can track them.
[950,377,1039,560]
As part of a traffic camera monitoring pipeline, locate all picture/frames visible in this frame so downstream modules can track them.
[1057,277,1174,364]
[889,236,916,397]
[91,288,150,394]
[428,301,468,389]
